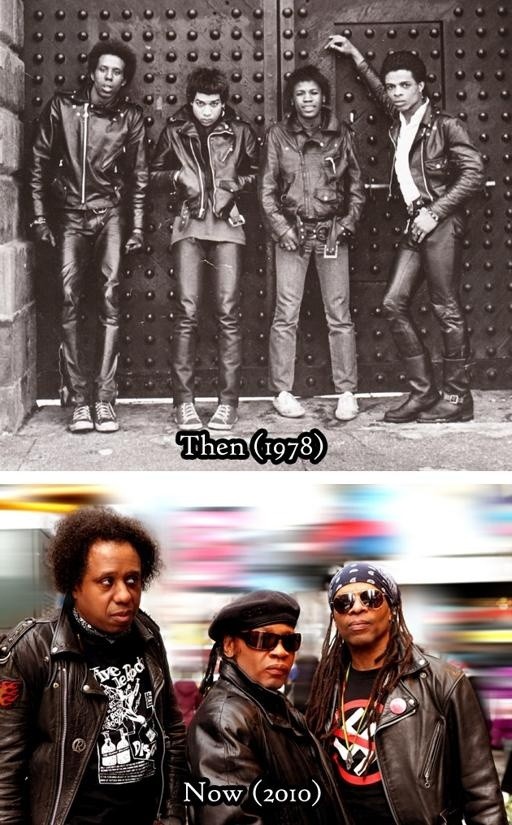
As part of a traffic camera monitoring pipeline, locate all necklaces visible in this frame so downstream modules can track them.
[338,656,394,775]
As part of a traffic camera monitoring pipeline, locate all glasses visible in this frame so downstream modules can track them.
[229,627,303,654]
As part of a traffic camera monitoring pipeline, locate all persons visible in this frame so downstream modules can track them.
[300,559,506,825]
[147,66,259,430]
[1,499,192,823]
[24,39,151,438]
[324,29,488,424]
[180,585,352,824]
[255,62,367,423]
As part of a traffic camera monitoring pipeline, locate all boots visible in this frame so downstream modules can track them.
[383,347,445,423]
[414,354,476,423]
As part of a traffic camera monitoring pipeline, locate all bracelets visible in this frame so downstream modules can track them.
[427,207,440,223]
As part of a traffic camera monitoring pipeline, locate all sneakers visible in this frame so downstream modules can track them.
[207,400,240,431]
[328,588,392,614]
[173,401,204,431]
[68,403,94,432]
[271,389,306,418]
[334,390,360,422]
[92,400,120,433]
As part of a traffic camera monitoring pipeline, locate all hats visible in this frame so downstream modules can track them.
[208,587,301,641]
